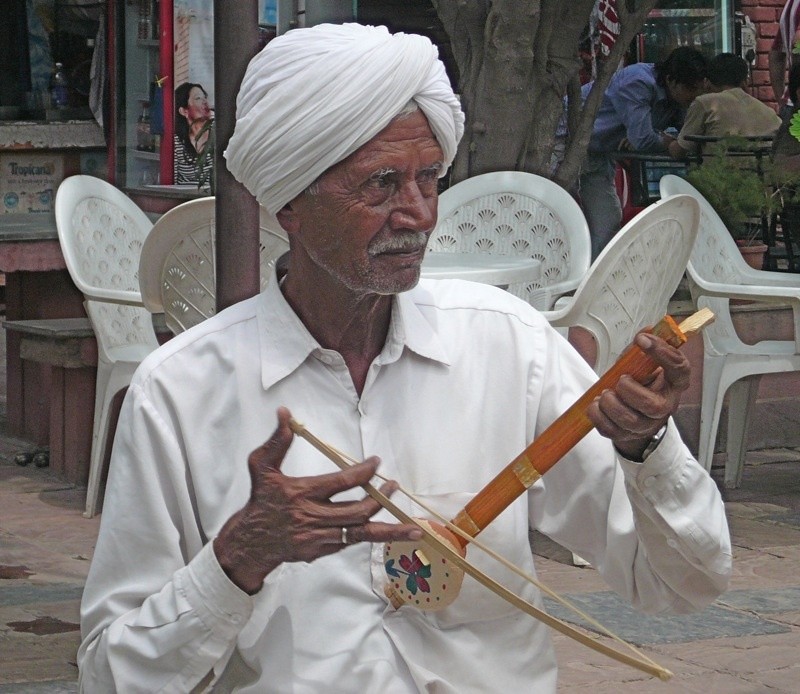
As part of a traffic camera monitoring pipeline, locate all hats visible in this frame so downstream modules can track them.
[225,20,467,220]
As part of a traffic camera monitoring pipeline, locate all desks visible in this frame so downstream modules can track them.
[602,148,698,213]
[418,248,545,294]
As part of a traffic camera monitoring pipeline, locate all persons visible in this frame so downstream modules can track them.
[76,23,733,694]
[550,45,707,268]
[669,52,783,238]
[172,83,213,188]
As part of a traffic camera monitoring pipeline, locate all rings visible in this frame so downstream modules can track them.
[341,525,347,545]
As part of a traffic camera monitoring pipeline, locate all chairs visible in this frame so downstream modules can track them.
[524,190,705,568]
[53,173,161,521]
[682,134,778,241]
[431,162,593,310]
[138,193,289,342]
[658,171,800,492]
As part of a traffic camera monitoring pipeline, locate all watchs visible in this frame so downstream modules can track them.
[640,424,668,462]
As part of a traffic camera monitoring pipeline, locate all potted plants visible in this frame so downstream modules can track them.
[671,131,800,304]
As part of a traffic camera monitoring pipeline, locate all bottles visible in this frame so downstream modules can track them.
[644,24,715,64]
[137,102,152,151]
[50,62,70,111]
